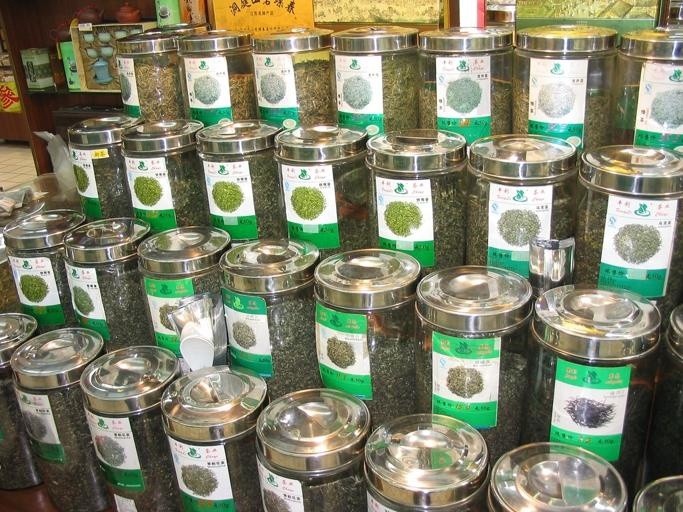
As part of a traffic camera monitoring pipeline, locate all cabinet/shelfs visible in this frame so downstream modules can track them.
[0,0,157,177]
